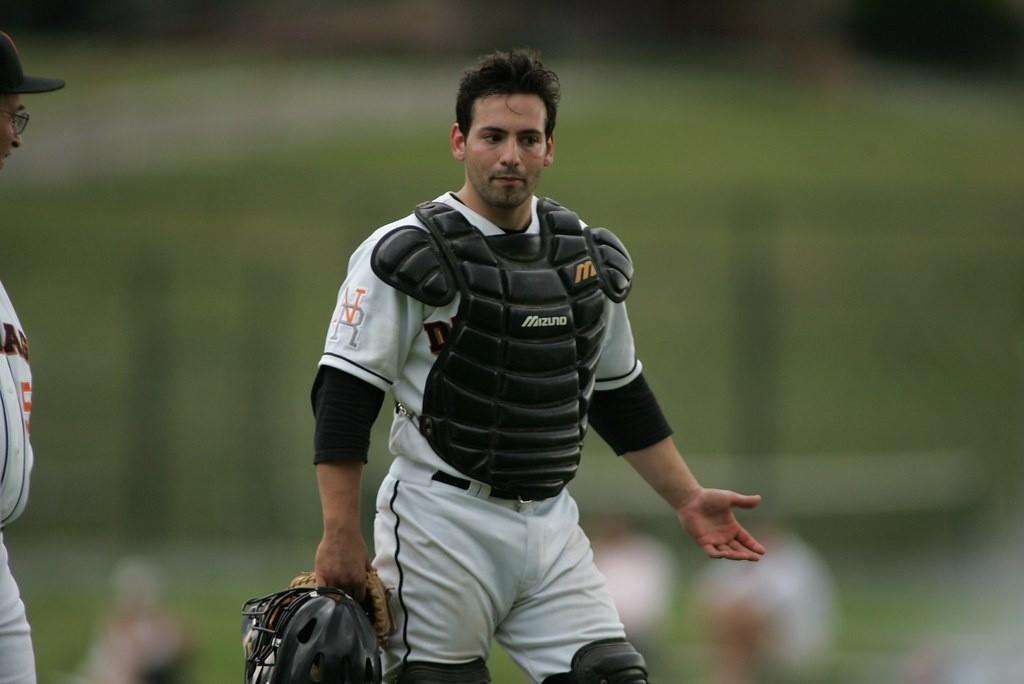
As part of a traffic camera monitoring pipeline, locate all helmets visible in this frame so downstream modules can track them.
[242,589,382,684]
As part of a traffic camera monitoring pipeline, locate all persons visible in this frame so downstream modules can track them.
[0,33,66,684]
[311,52,767,684]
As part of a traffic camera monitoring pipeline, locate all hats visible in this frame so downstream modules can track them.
[0,31,65,93]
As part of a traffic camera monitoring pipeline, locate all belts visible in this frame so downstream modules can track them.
[431,470,544,503]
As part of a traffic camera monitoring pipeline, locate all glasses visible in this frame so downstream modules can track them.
[0,109,30,134]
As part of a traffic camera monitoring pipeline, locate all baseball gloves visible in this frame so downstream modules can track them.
[293,564,398,640]
